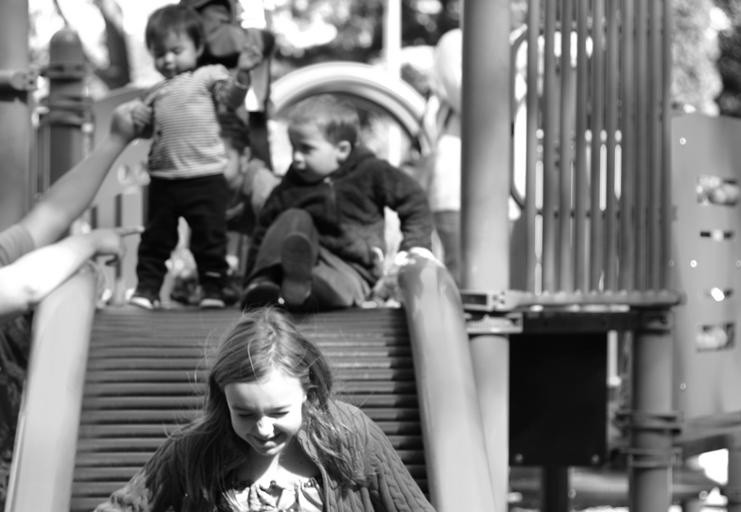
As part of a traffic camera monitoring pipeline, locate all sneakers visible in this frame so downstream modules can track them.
[197,272,228,309]
[127,282,161,311]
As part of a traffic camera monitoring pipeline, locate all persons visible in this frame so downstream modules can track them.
[167,1,289,308]
[90,305,440,512]
[1,98,146,512]
[122,4,255,310]
[240,90,432,310]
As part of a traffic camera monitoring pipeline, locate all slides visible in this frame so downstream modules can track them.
[5,257,493,512]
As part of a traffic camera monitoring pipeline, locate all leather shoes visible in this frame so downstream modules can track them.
[239,232,312,312]
